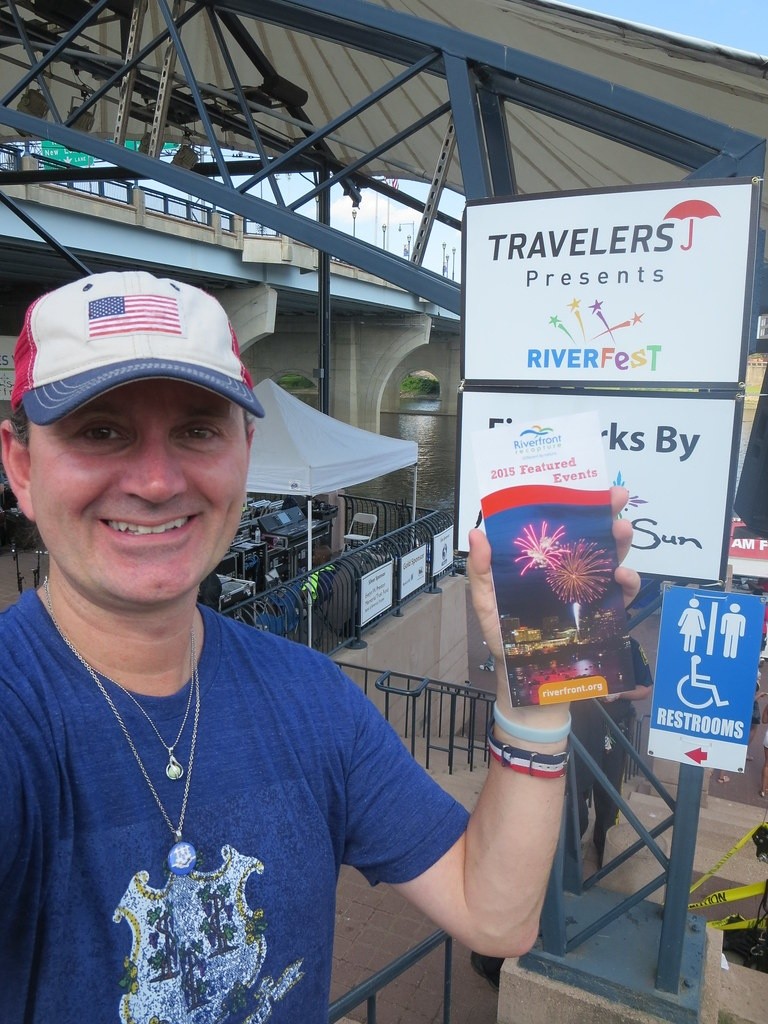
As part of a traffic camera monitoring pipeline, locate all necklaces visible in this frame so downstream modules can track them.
[46,575,200,875]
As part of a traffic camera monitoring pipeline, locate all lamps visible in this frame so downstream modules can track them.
[14,84,198,172]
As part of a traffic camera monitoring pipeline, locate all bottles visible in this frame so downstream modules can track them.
[255,527,261,543]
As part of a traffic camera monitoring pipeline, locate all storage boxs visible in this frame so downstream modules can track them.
[195,532,330,628]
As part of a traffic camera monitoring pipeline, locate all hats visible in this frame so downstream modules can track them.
[11,271,265,426]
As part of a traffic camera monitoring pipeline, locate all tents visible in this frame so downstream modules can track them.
[245,377,419,648]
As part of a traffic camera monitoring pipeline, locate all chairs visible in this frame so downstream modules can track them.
[339,512,377,568]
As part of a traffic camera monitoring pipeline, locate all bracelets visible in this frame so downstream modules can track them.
[488,728,570,778]
[492,705,572,743]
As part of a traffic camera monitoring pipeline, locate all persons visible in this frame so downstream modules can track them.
[0,271,641,1024]
[711,658,768,796]
[569,632,652,872]
